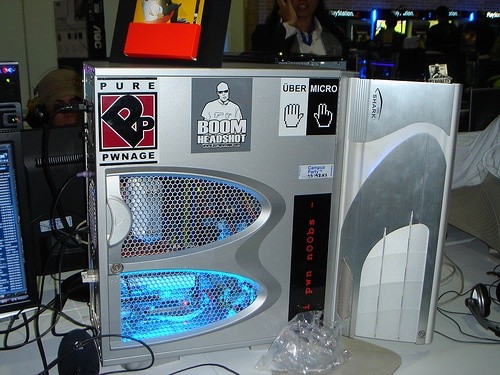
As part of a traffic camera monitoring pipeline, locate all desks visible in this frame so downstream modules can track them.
[0,239,500,375]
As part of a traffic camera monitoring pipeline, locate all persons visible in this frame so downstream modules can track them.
[427,6,461,51]
[251,0,350,59]
[460,10,497,59]
[373,13,406,50]
[36,69,84,128]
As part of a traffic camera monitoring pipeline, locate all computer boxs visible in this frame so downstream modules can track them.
[82,61,463,365]
[21,125,86,275]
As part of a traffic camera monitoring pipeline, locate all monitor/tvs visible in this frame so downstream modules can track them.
[374,20,406,36]
[0,133,40,313]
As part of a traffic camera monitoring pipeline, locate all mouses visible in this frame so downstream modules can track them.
[58,329,99,375]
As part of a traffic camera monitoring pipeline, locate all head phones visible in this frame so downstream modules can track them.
[465,281,500,338]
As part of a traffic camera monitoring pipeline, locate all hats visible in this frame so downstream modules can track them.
[38,69,85,102]
